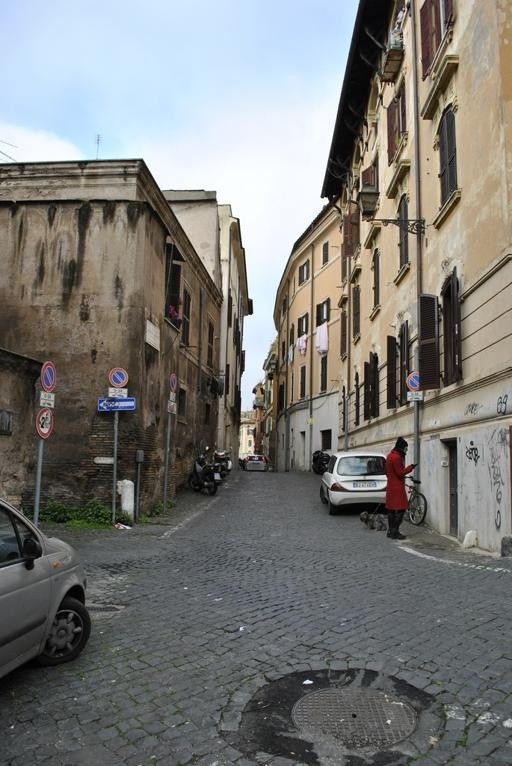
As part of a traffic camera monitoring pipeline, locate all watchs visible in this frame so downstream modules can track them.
[190,445,222,497]
[214,449,232,476]
[311,448,330,474]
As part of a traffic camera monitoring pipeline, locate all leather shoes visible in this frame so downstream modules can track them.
[411,463,416,468]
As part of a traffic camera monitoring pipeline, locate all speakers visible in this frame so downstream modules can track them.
[387,518,406,539]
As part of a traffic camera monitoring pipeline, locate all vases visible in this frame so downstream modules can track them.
[168,295,184,322]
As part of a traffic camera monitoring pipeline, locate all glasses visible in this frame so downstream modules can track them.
[355,180,426,235]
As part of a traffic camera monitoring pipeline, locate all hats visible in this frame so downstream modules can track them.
[0,497,92,688]
[318,452,390,514]
[243,455,267,471]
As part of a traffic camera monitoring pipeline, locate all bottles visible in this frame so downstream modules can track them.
[396,437,408,451]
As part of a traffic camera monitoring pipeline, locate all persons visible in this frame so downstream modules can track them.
[384,438,418,539]
